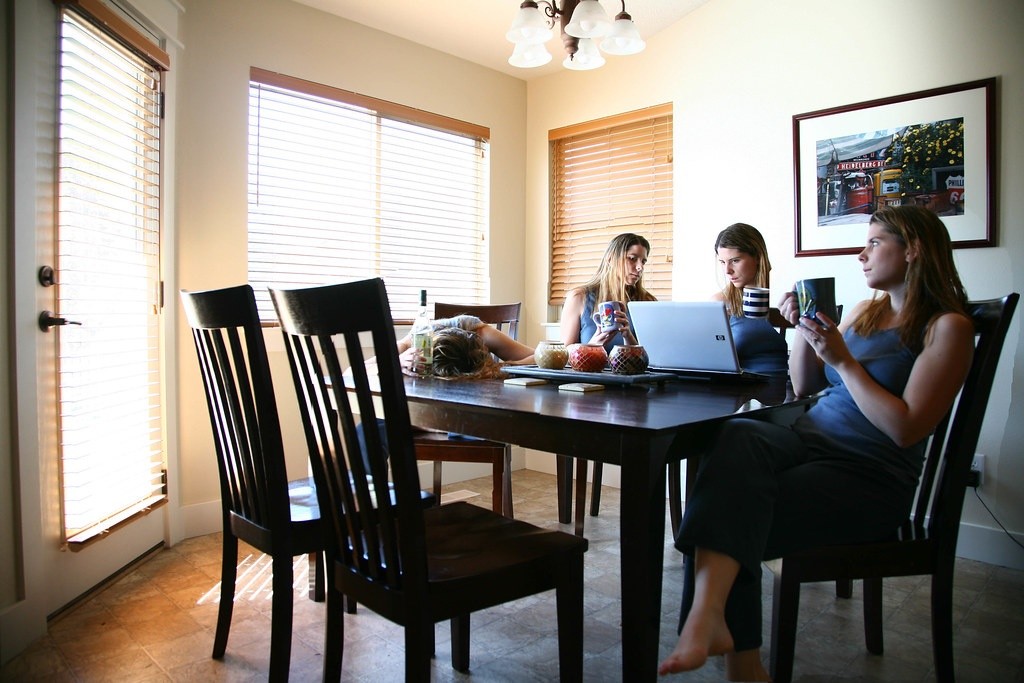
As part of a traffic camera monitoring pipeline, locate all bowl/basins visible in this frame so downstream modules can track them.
[535,341,649,375]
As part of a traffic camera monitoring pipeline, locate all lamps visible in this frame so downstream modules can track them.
[507,0,645,71]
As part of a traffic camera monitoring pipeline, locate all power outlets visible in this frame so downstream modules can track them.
[971,452,987,486]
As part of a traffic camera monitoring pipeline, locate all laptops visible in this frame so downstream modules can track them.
[627,301,775,379]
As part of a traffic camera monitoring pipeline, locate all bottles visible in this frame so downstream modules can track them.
[412,288,434,376]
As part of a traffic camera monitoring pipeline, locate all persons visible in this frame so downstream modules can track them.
[658,204,975,683]
[558,233,659,366]
[345,316,538,475]
[708,222,789,376]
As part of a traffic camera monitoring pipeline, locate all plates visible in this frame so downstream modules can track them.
[501,365,674,386]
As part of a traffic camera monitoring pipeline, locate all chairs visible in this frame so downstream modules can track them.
[178,284,440,683]
[413,303,522,520]
[556,455,682,542]
[770,305,843,412]
[267,276,589,683]
[770,292,1021,683]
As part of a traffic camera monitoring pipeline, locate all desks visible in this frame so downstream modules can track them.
[307,363,822,683]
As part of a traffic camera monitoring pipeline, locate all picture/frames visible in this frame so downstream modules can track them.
[791,76,997,257]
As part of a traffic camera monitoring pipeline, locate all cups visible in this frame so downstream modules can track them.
[793,277,839,328]
[742,287,770,319]
[592,300,624,332]
